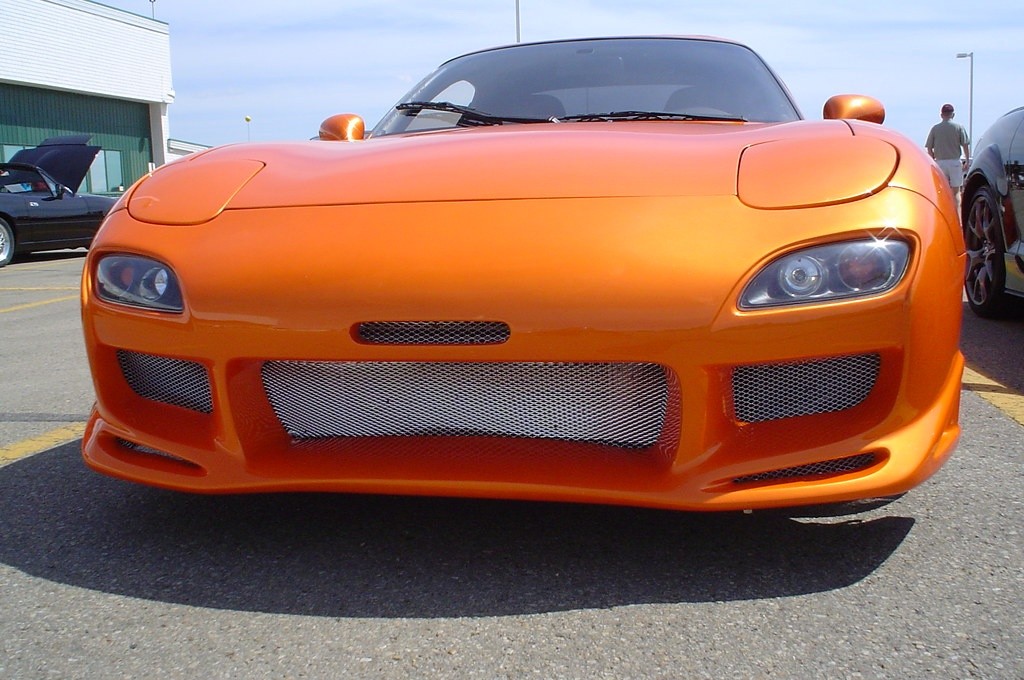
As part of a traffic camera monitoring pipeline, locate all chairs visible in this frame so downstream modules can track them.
[664,85,745,117]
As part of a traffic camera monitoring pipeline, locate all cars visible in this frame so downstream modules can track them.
[958,106,1024,321]
[0,133,120,269]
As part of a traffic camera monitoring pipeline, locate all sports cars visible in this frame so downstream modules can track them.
[79,34,966,514]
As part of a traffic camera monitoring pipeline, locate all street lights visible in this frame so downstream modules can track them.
[957,52,975,159]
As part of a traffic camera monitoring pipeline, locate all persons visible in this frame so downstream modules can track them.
[925,104,970,227]
